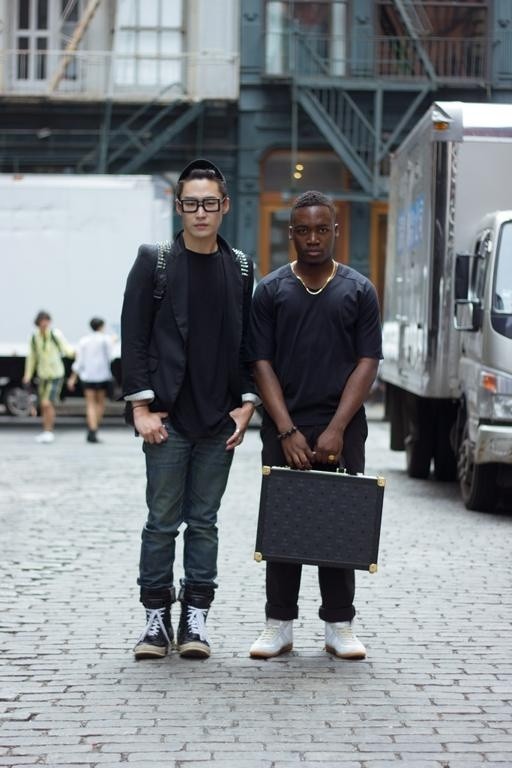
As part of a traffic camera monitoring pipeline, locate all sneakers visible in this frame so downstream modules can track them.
[325,620,367,660]
[36,430,98,443]
[250,617,293,658]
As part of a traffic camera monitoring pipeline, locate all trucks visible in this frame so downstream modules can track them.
[377,100,511,511]
[0,173,174,418]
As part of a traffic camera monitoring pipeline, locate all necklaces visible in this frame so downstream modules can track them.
[292,258,337,298]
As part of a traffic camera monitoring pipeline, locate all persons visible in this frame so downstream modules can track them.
[242,187,385,662]
[120,156,267,651]
[68,319,112,442]
[19,311,76,444]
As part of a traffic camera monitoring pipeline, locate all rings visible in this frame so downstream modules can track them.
[328,454,335,461]
[237,438,242,441]
[235,429,241,434]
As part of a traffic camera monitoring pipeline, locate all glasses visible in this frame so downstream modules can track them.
[179,199,224,213]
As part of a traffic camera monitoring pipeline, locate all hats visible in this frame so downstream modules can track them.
[178,159,226,181]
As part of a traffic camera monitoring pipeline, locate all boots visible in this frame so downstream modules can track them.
[176,603,211,658]
[133,607,175,659]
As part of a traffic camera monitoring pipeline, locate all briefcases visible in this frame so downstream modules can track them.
[254,466,386,574]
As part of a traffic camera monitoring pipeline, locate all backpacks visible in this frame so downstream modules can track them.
[110,240,249,439]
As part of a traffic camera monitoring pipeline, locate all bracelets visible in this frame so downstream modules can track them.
[133,404,148,409]
[277,426,298,440]
